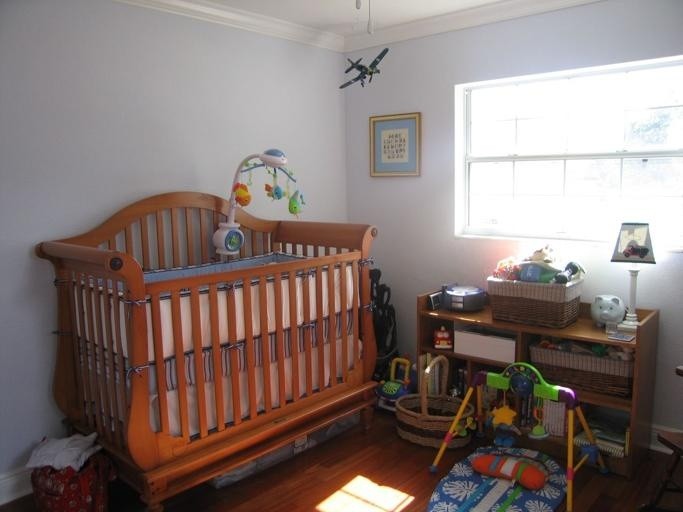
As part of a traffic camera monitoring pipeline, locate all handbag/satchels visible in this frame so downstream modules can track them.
[31,437,118,512]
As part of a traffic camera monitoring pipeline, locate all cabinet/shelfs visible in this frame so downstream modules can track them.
[413,289,658,481]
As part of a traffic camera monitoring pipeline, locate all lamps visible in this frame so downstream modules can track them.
[610,222,657,332]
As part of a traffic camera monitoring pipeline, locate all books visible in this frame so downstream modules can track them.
[572,417,626,460]
[523,393,569,438]
[418,351,469,399]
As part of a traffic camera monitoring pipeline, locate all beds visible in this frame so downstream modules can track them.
[31,187,386,511]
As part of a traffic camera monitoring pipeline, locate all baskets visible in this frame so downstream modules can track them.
[487,275,585,329]
[394,355,474,449]
[528,340,634,397]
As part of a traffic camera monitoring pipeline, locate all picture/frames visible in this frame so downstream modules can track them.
[368,112,421,176]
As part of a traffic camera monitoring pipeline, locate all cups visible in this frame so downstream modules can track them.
[605,321,620,337]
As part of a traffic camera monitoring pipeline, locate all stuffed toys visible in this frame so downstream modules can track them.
[489,244,587,284]
